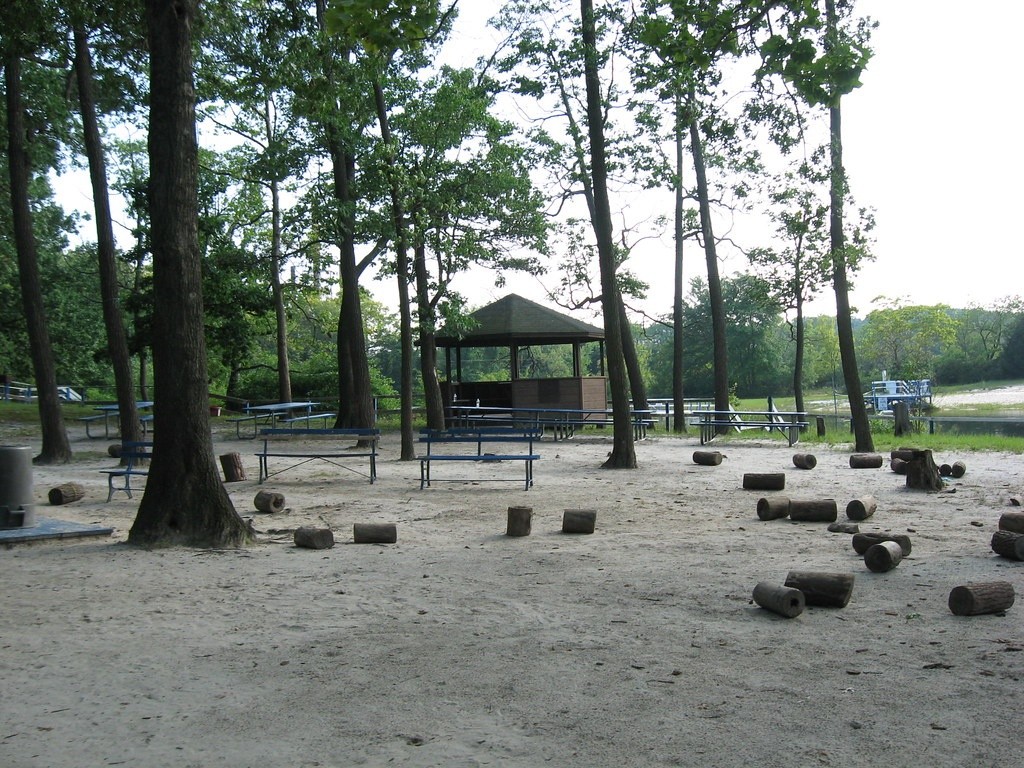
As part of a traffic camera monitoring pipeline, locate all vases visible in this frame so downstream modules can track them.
[209,406,222,416]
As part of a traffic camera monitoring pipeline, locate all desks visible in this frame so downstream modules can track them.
[692,410,808,444]
[608,397,715,411]
[445,405,656,441]
[242,402,321,436]
[93,402,155,440]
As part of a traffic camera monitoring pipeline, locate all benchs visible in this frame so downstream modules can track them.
[99,442,154,504]
[413,427,541,491]
[253,429,381,485]
[139,414,154,439]
[277,413,337,436]
[445,417,649,443]
[78,412,120,440]
[690,422,805,447]
[701,419,810,443]
[458,414,660,440]
[226,412,289,439]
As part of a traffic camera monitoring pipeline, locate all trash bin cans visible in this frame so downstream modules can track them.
[0,443,37,531]
